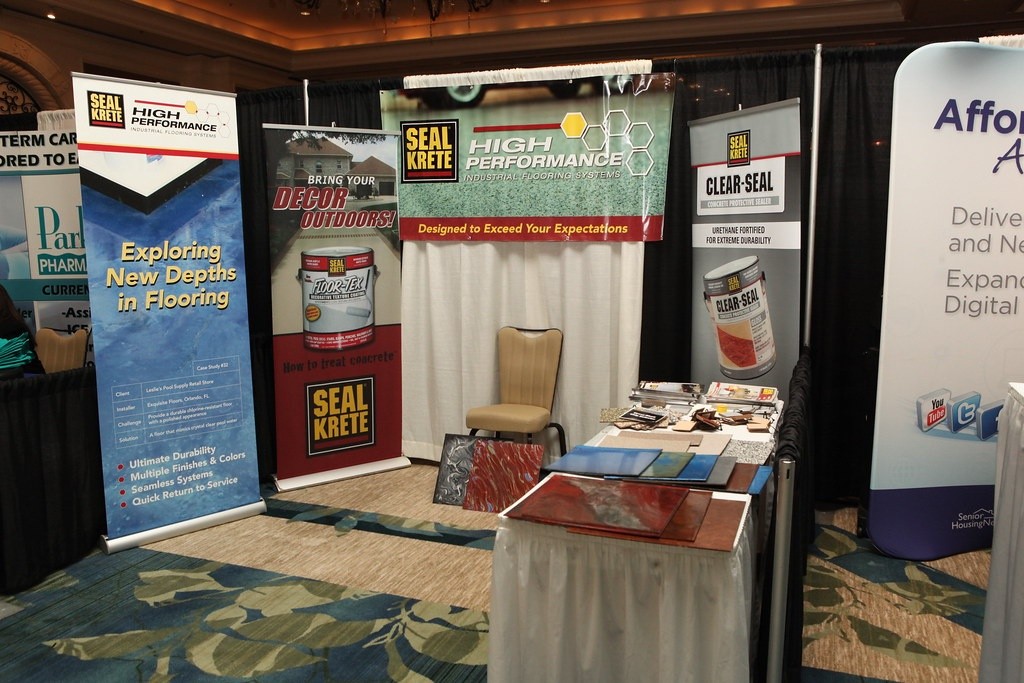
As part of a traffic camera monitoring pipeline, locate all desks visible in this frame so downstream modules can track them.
[488,401,788,683]
[0,367,107,596]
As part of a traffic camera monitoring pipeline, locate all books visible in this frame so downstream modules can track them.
[703,382,779,406]
[629,381,701,406]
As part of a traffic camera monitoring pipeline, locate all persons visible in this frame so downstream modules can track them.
[0,284,46,374]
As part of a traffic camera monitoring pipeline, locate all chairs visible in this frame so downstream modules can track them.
[466,326,567,456]
[35,326,90,375]
